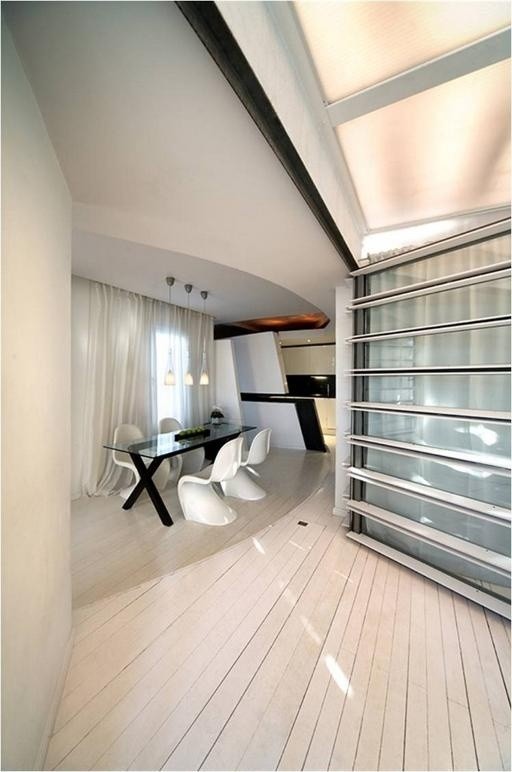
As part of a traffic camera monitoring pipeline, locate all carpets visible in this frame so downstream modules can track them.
[68,447,331,602]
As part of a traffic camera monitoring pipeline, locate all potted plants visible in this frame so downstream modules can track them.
[211,411,225,425]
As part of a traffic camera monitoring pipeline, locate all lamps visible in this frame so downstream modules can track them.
[163,277,210,388]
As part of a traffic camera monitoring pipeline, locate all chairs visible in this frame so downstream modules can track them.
[113,418,209,503]
[176,427,273,527]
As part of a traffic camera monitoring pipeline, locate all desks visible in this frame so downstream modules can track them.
[103,423,257,527]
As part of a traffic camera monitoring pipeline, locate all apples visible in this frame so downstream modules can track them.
[179,427,206,435]
